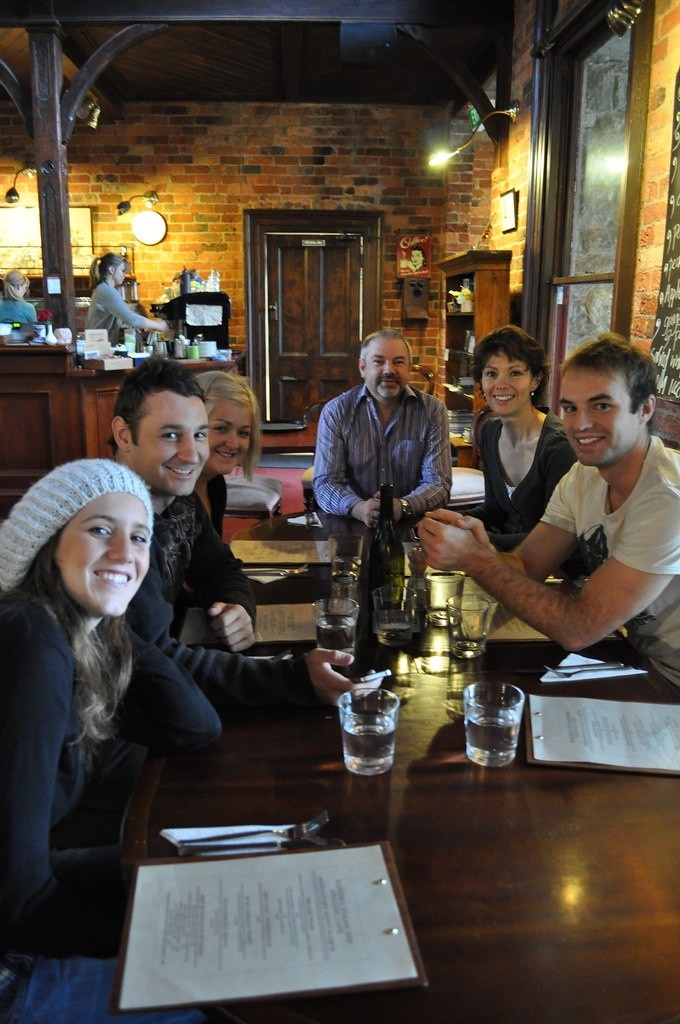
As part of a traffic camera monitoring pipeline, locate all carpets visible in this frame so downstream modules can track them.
[253,452,313,470]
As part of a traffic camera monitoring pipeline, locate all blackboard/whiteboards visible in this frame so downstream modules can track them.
[643,67,680,407]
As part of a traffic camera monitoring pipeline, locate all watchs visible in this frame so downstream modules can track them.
[399,498,412,524]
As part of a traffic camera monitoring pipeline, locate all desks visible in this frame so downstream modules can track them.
[110,497,680,1023]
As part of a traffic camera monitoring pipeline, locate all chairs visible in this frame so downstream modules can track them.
[223,475,283,523]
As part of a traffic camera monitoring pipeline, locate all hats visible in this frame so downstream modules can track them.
[0,458,154,593]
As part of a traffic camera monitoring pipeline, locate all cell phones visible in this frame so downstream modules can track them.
[347,669,391,683]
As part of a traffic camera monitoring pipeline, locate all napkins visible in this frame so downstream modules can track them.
[287,510,324,528]
[158,825,317,856]
[540,653,646,682]
[247,572,287,585]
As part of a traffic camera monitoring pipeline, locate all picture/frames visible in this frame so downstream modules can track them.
[500,187,517,233]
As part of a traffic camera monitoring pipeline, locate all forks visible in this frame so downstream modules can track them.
[177,810,330,846]
[242,564,309,573]
[543,662,632,679]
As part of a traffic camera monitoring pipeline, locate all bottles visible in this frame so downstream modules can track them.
[405,549,430,610]
[45,325,57,345]
[181,266,190,295]
[368,485,404,609]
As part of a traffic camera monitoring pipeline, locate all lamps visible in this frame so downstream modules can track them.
[117,191,159,216]
[430,100,517,169]
[609,1,648,39]
[5,159,39,203]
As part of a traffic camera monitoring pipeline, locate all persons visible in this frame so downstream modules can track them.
[0,461,222,1023]
[104,360,357,716]
[193,372,322,605]
[312,327,453,536]
[85,252,169,347]
[474,325,579,554]
[0,270,37,323]
[417,331,679,692]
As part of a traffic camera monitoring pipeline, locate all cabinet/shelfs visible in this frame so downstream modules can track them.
[439,248,513,468]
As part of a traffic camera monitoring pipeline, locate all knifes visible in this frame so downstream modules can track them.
[178,837,343,856]
[510,663,624,672]
[244,569,309,577]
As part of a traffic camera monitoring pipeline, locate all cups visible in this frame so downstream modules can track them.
[373,585,418,647]
[462,681,526,767]
[329,533,363,586]
[312,596,359,655]
[444,594,492,659]
[424,571,466,627]
[338,687,403,775]
[32,325,46,343]
